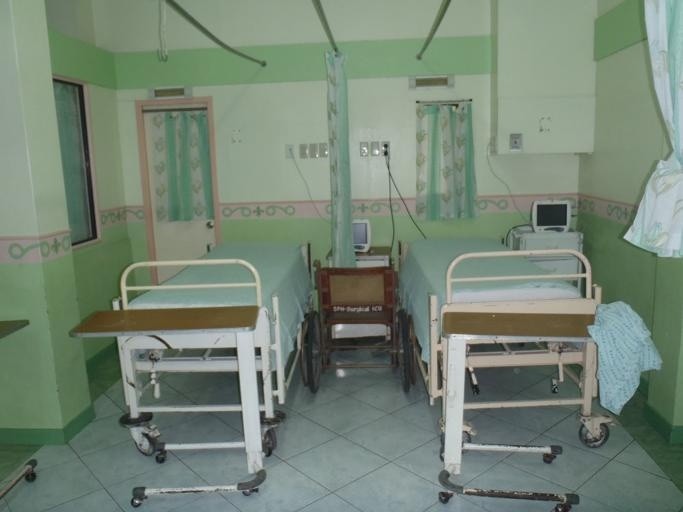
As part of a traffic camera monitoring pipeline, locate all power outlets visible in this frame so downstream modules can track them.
[285,144,296,159]
[381,141,390,157]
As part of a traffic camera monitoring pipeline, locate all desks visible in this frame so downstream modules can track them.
[437,309,603,511]
[69,308,272,508]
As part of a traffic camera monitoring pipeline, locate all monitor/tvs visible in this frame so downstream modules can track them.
[352,219,372,253]
[532,200,570,232]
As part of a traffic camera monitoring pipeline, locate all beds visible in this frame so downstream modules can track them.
[120,241,312,407]
[397,237,617,415]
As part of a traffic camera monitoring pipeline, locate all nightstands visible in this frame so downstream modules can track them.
[511,228,584,289]
[326,241,391,269]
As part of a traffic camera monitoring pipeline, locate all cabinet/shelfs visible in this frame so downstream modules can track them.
[489,1,598,156]
